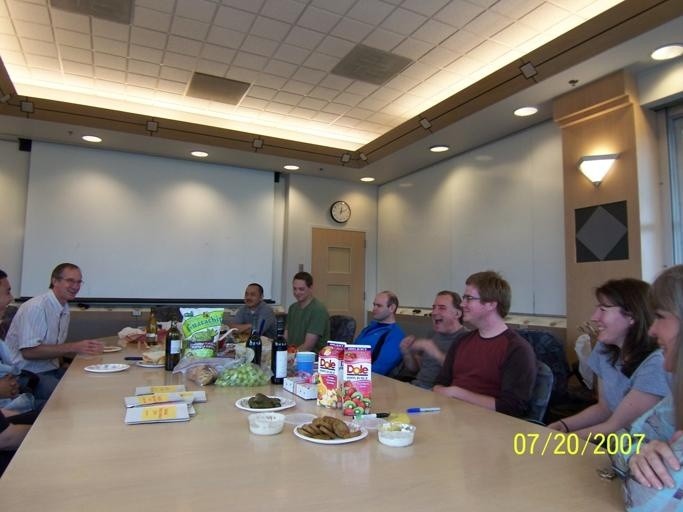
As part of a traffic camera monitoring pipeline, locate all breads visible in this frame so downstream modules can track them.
[141,351,166,365]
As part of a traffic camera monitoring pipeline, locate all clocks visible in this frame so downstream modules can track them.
[330,201,351,223]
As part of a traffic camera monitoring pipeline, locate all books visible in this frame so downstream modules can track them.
[135,385,187,394]
[123,393,184,408]
[125,407,189,423]
[187,404,196,418]
[177,390,208,404]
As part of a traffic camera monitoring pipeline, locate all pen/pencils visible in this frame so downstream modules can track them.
[407,406,441,413]
[124,357,143,360]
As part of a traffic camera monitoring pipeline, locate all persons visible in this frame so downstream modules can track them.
[398,290,472,391]
[220,281,278,340]
[426,269,539,420]
[5,263,106,404]
[282,271,331,361]
[351,290,406,376]
[544,277,675,447]
[0,407,42,478]
[604,261,682,512]
[0,270,58,414]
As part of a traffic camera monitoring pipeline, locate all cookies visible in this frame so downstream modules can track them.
[297,416,360,439]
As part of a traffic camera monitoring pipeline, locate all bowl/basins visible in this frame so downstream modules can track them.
[247,413,285,434]
[376,421,417,448]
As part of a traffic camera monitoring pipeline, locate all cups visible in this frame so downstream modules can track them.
[295,351,315,376]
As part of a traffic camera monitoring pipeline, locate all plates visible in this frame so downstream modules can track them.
[353,416,387,429]
[286,415,316,424]
[135,361,164,368]
[102,347,121,353]
[294,422,368,447]
[234,396,296,413]
[82,364,130,373]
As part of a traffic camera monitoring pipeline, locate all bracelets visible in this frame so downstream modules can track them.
[559,419,569,433]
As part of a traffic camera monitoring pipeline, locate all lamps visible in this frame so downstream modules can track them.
[576,155,618,189]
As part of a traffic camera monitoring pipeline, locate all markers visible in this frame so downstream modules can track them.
[353,413,389,420]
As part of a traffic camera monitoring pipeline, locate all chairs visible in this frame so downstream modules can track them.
[521,361,553,426]
[276,312,287,337]
[515,328,598,424]
[330,315,357,343]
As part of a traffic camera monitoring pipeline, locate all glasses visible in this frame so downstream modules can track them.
[462,294,481,302]
[55,276,85,287]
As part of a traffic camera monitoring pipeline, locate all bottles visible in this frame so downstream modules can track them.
[165,314,182,371]
[246,314,261,366]
[272,313,288,384]
[148,309,157,334]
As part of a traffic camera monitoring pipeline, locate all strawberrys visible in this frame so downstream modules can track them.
[343,381,371,415]
